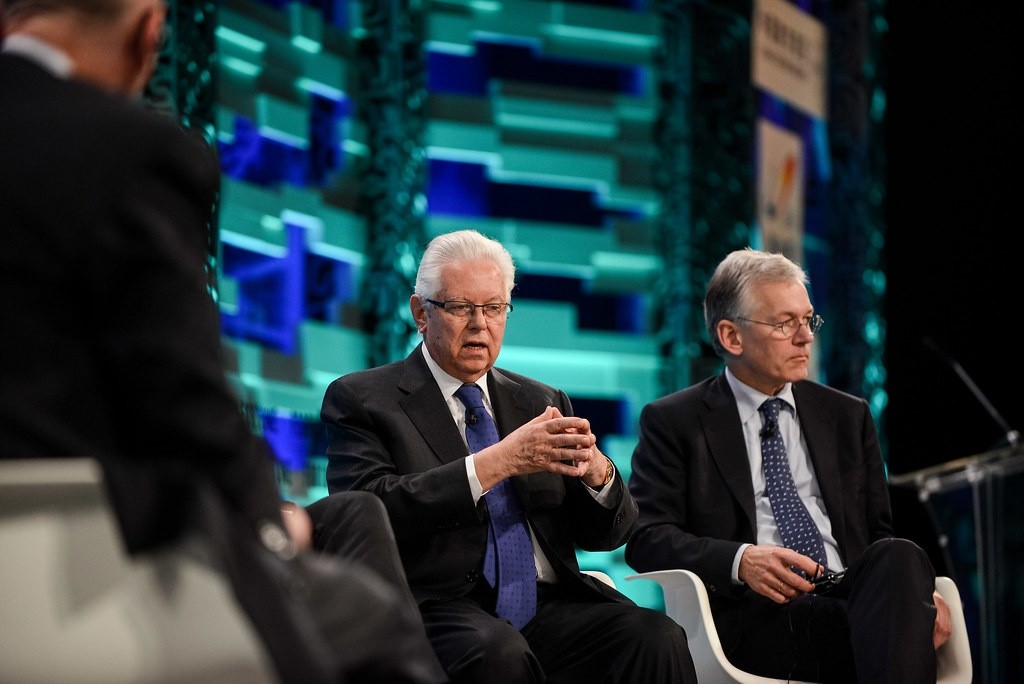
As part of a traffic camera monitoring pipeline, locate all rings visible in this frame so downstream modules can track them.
[778,582,784,592]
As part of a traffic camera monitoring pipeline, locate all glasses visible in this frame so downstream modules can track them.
[731,314,825,337]
[425,299,513,318]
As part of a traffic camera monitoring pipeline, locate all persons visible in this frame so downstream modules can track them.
[319,229,697,684]
[623,246,952,684]
[0,0,449,684]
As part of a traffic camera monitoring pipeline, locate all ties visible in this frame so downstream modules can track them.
[758,398,828,580]
[452,382,537,632]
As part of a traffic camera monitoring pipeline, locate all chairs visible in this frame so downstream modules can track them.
[624,566,975,684]
[0,459,273,684]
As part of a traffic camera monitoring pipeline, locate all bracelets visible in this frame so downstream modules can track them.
[604,457,613,486]
